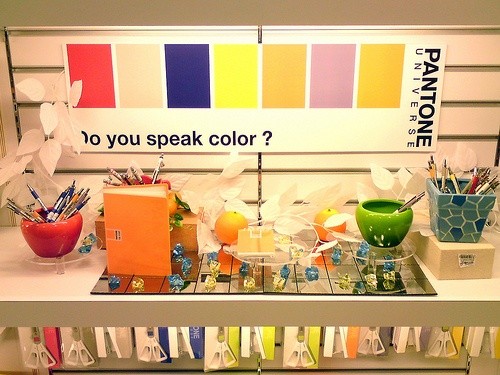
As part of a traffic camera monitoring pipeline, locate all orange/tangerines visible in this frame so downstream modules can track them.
[314,208,346,241]
[313,241,336,270]
[215,211,248,244]
[217,243,243,275]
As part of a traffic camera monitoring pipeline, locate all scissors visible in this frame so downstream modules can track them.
[358,326,385,354]
[138,327,168,362]
[286,326,315,367]
[25,327,56,369]
[209,327,236,369]
[428,331,458,356]
[66,327,95,366]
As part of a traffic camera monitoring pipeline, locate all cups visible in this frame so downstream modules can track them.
[426,177,496,242]
[20,206,84,258]
[355,198,413,248]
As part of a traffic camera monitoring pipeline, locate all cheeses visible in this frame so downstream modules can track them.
[237,226,276,256]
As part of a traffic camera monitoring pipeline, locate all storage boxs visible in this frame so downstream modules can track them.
[95,209,199,255]
[425,174,497,243]
[412,222,495,281]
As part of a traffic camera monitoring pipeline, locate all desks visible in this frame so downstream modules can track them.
[0,168,500,373]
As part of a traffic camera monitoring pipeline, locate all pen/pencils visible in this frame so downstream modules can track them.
[27,184,50,214]
[103,179,122,186]
[130,167,145,185]
[461,165,500,195]
[425,156,440,191]
[7,198,46,223]
[448,167,461,194]
[443,160,445,191]
[393,191,425,212]
[65,197,91,219]
[46,180,90,222]
[152,153,164,184]
[106,167,122,181]
[120,173,136,185]
